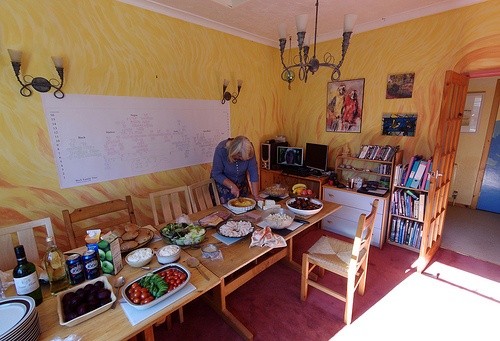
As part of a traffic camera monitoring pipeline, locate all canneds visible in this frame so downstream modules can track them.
[82,249,101,280]
[66,254,86,285]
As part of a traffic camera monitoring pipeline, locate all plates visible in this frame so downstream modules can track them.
[216,218,254,238]
[120,263,192,307]
[55,275,116,327]
[199,209,230,231]
[227,197,256,212]
[119,228,155,252]
[0,296,39,341]
[160,223,207,246]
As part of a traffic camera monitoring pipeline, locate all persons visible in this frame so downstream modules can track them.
[209,135,259,206]
[280,148,298,165]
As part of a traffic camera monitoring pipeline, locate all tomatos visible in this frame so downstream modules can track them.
[161,269,186,292]
[128,282,154,305]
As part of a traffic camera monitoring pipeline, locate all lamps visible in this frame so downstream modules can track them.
[7,49,65,99]
[221,79,243,104]
[277,0,359,84]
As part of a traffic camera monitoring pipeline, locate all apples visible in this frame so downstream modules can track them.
[307,190,312,194]
[301,190,307,196]
[98,241,114,273]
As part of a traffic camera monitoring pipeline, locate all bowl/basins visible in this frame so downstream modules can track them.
[262,207,294,230]
[286,197,324,216]
[125,247,153,268]
[156,245,181,264]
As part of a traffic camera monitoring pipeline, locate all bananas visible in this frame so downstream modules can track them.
[292,184,307,194]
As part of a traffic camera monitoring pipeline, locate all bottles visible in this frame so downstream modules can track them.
[43,236,69,295]
[12,244,42,306]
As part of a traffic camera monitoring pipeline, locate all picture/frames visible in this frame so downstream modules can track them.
[327,77,365,133]
[459,90,486,136]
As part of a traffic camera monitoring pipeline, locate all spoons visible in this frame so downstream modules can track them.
[185,257,211,281]
[111,275,126,310]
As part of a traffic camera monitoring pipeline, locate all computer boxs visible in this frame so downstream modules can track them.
[260,142,289,170]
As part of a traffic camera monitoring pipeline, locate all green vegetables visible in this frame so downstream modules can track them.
[138,272,168,300]
[161,223,204,241]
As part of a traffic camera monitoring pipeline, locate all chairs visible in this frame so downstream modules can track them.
[188,178,221,214]
[148,185,192,226]
[0,194,183,341]
[300,198,379,326]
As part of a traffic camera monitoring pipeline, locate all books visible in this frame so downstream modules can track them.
[391,189,428,221]
[390,217,423,249]
[356,144,400,161]
[394,155,434,191]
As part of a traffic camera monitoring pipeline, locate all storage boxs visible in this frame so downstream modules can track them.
[85,228,103,269]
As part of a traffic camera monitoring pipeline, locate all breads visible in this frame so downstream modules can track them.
[112,222,151,251]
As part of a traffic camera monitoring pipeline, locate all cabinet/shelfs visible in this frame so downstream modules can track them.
[335,150,395,192]
[386,150,430,253]
[321,183,390,249]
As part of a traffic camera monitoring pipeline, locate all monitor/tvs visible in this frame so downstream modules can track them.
[306,143,328,176]
[276,146,304,169]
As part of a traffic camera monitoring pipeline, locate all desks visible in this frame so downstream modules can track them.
[153,192,343,341]
[0,225,220,341]
[260,167,329,228]
